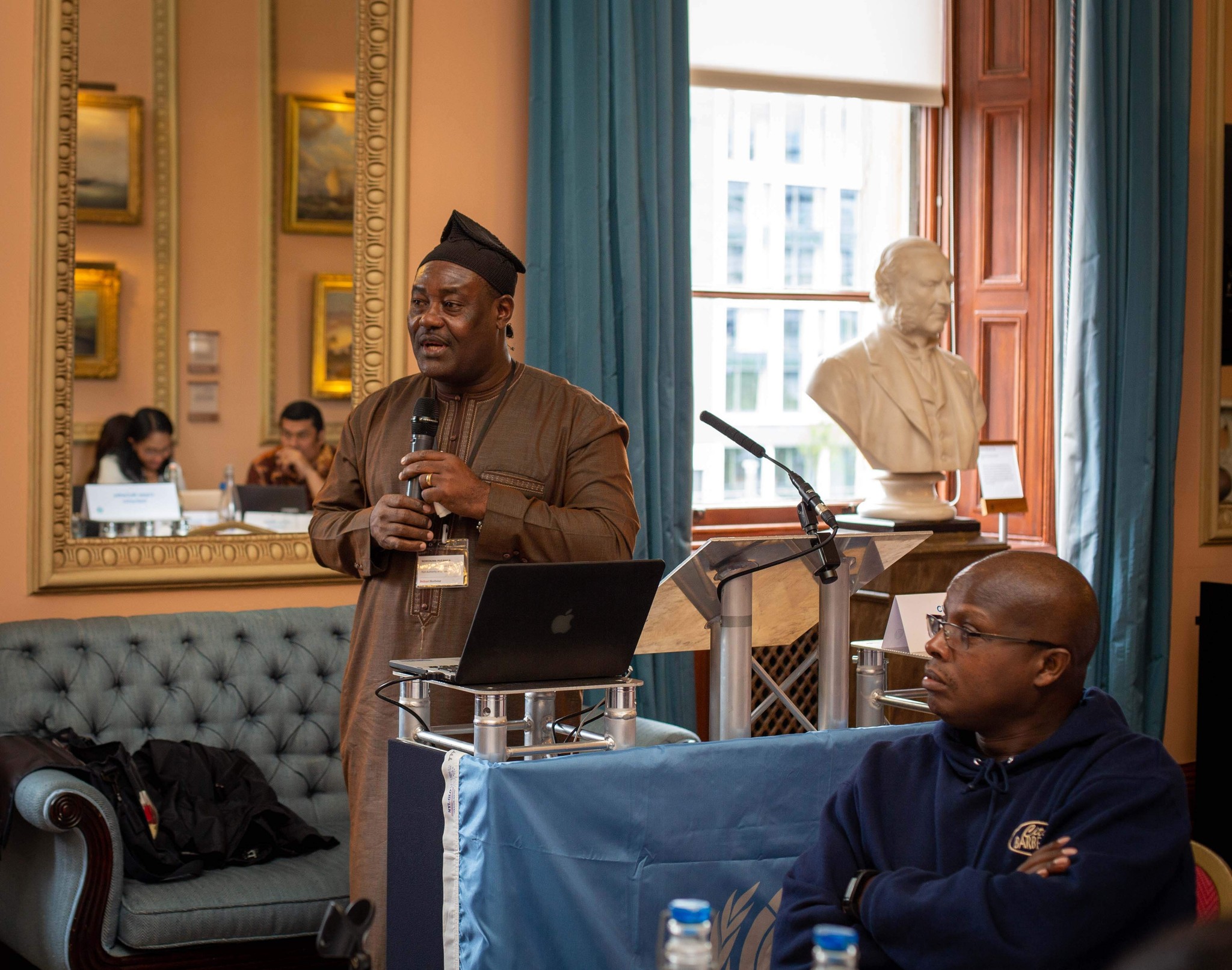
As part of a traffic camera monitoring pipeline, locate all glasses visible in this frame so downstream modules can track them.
[927,614,1058,653]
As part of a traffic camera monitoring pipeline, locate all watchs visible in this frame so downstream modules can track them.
[841,869,880,923]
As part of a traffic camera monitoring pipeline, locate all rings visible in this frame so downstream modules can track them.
[426,473,433,487]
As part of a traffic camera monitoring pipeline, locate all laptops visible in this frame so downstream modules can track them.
[236,484,309,514]
[388,558,666,686]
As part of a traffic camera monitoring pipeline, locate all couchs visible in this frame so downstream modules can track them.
[0,605,691,970]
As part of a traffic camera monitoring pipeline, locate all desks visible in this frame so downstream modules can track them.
[392,670,644,765]
[851,639,934,726]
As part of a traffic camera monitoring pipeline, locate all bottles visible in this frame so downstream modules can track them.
[218,467,241,533]
[662,898,721,970]
[809,923,857,970]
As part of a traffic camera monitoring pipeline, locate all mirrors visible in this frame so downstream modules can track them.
[25,0,413,597]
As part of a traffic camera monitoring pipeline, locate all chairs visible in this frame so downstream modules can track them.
[1189,840,1232,924]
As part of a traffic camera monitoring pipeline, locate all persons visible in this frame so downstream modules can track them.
[770,551,1197,970]
[246,401,337,510]
[308,209,643,970]
[804,236,987,473]
[98,408,186,493]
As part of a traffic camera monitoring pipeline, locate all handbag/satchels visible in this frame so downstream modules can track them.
[0,727,181,884]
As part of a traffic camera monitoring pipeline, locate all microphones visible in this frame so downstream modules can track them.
[404,397,441,502]
[701,410,838,529]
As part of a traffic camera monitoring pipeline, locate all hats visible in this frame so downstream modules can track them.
[418,208,526,298]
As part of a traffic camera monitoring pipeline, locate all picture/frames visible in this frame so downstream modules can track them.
[71,268,120,378]
[1198,0,1232,546]
[311,276,355,397]
[69,89,143,226]
[280,95,357,235]
